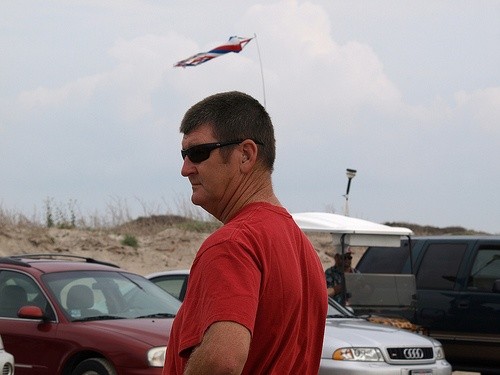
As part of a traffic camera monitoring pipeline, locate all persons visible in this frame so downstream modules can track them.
[162,91,329,375]
[326,244,359,304]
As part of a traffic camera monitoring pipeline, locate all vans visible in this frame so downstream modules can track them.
[353,235,500,375]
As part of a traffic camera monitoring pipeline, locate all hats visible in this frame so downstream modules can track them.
[334,246,354,258]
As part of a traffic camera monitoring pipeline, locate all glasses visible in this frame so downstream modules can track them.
[180,141,266,164]
[338,256,352,260]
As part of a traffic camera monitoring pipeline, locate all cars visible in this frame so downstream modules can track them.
[96,269,455,375]
[1,251,185,375]
